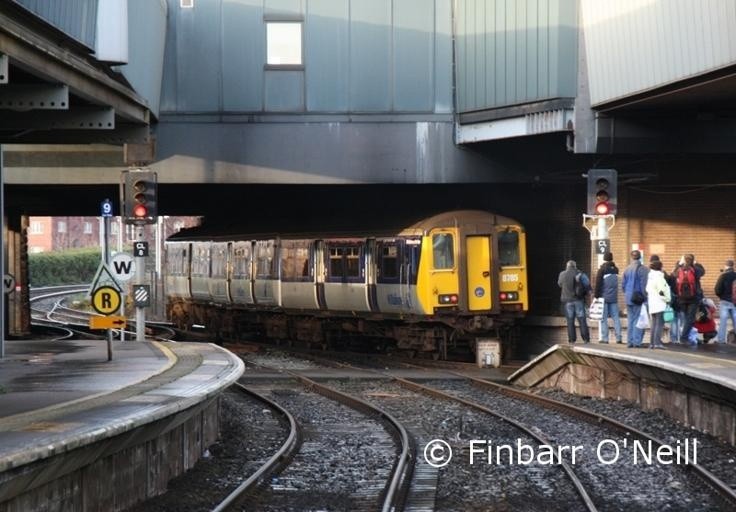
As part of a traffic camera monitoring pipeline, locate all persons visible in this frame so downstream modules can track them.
[557,250,736,349]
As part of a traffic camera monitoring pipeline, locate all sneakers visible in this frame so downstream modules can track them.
[629,340,681,348]
[570,337,622,343]
[715,340,726,344]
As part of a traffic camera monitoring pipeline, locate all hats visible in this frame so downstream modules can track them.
[604,253,612,261]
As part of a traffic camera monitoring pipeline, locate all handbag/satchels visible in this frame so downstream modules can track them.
[631,291,644,304]
[574,272,587,297]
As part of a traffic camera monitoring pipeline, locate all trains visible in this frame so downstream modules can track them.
[166,210,530,361]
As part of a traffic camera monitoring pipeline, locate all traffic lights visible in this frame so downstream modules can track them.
[588,169,616,217]
[121,171,158,225]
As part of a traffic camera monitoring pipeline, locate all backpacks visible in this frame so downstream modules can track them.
[676,265,696,297]
[695,304,712,322]
